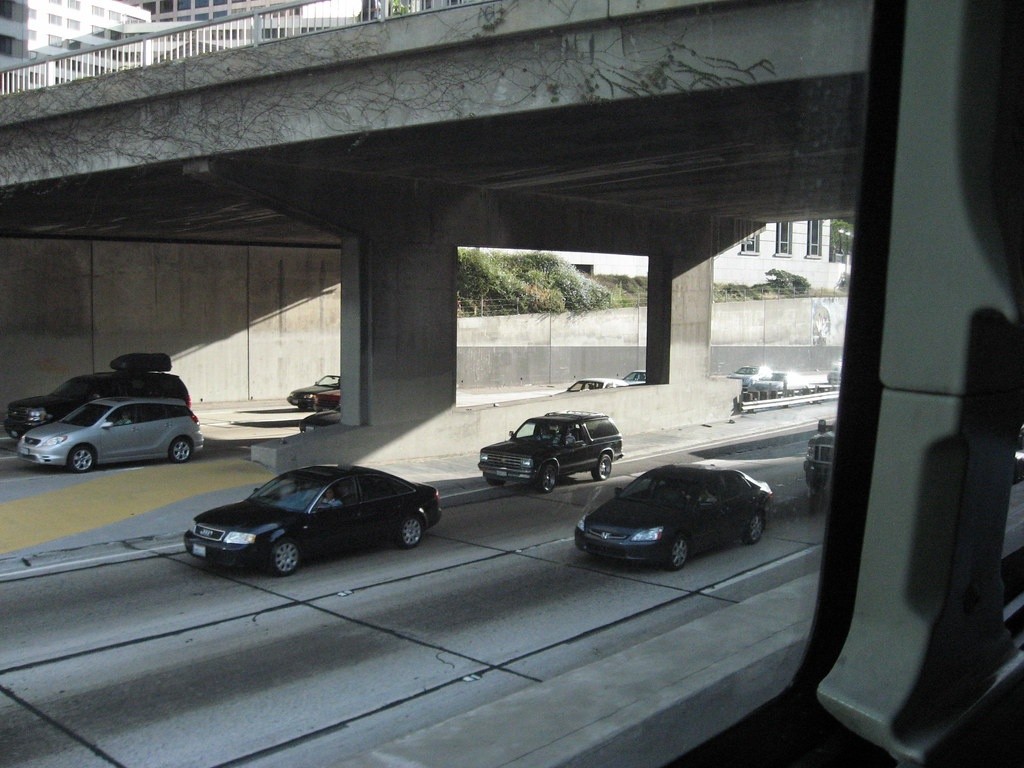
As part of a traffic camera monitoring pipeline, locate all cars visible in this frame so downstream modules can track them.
[554,376,630,397]
[996,448,1024,568]
[748,371,808,395]
[298,410,340,434]
[623,370,647,386]
[312,386,342,413]
[727,363,773,391]
[287,372,341,411]
[825,362,842,385]
[574,463,774,573]
[183,463,443,577]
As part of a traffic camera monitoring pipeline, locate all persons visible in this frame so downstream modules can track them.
[552,425,575,447]
[684,486,718,505]
[535,424,553,441]
[113,409,132,425]
[322,487,345,507]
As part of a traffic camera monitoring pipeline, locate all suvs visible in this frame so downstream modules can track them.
[803,420,834,493]
[15,397,204,472]
[478,411,625,493]
[4,352,192,444]
